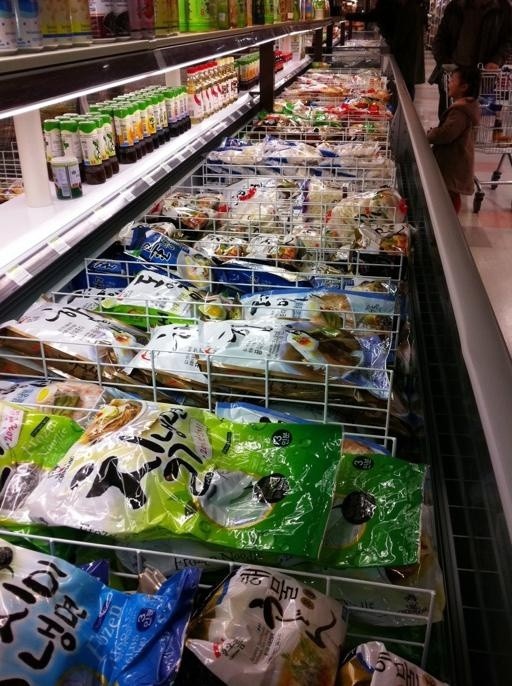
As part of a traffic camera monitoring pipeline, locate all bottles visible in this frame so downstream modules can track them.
[0,1,330,60]
[492,110,503,143]
[40,52,264,184]
[273,47,295,72]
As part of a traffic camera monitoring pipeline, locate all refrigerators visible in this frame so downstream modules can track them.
[1,26,512,686]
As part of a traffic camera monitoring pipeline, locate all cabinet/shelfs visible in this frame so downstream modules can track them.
[0,13,339,314]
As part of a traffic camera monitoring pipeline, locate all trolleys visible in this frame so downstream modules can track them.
[442,60,512,216]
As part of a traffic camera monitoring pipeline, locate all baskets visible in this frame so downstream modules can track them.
[22,273,403,390]
[143,67,399,226]
[81,231,405,302]
[25,377,399,583]
[0,529,442,686]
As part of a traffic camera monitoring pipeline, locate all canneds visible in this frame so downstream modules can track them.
[51,156,83,199]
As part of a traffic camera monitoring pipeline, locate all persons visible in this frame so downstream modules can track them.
[435,0,510,131]
[427,61,484,215]
[344,0,426,103]
